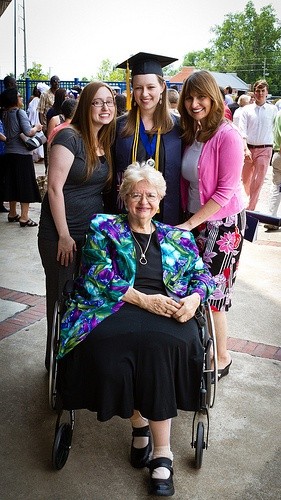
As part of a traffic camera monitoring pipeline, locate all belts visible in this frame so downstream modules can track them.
[247,144,273,148]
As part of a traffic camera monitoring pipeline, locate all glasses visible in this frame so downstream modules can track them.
[90,97,116,107]
[52,80,60,83]
[18,95,22,98]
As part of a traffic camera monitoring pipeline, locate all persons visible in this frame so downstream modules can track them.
[37,81,117,377]
[109,52,184,226]
[173,70,250,384]
[220,78,281,228]
[0,75,81,227]
[112,85,182,119]
[55,161,216,495]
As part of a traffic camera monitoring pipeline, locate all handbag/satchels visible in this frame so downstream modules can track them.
[37,175,48,202]
[16,109,47,151]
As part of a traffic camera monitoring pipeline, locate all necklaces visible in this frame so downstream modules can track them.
[127,218,153,265]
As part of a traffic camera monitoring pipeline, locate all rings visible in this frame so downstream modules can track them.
[165,310,167,313]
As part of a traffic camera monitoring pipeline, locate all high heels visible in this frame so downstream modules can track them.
[8,213,21,222]
[209,358,232,383]
[19,218,38,227]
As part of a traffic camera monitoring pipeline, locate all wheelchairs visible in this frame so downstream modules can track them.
[43,245,218,471]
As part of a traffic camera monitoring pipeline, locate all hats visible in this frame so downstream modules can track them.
[116,52,179,111]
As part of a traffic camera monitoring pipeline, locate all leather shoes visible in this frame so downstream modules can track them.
[130,425,151,469]
[145,458,176,495]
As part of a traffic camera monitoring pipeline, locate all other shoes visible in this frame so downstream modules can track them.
[264,224,279,230]
